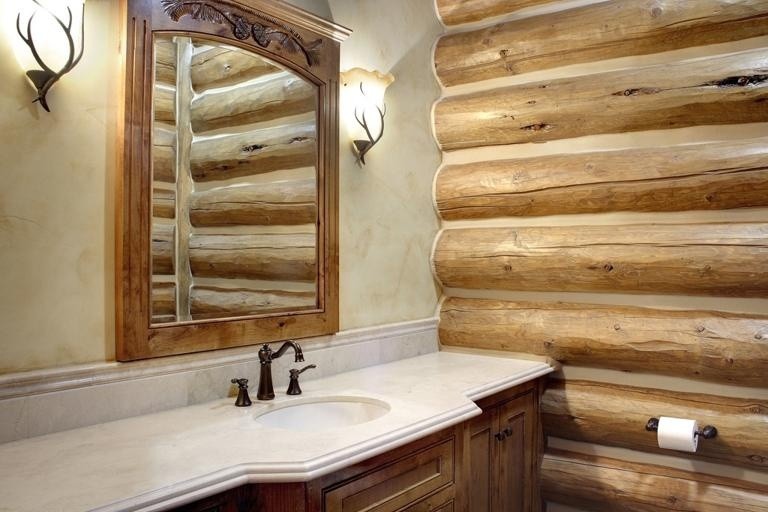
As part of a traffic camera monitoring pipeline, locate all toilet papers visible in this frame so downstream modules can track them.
[657,417,699,454]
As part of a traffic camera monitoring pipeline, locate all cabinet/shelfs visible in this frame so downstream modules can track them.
[305,425,464,512]
[464,380,544,512]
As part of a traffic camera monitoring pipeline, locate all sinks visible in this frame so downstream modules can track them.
[253,397,391,433]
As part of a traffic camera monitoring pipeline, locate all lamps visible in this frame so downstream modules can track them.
[338,68,395,165]
[6,0,86,113]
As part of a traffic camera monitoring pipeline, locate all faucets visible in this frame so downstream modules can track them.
[257,340,304,400]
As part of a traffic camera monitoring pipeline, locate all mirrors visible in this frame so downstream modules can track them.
[114,0,353,363]
